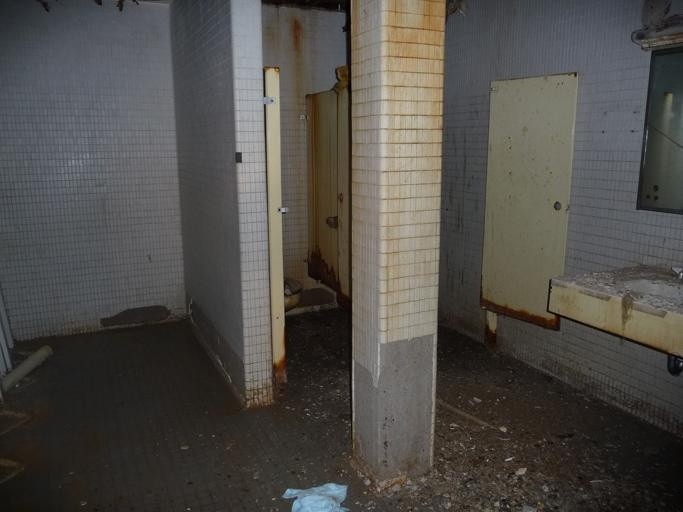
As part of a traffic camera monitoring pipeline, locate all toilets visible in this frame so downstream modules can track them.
[284,278,303,312]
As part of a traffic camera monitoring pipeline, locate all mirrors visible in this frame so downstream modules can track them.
[636,43,683,215]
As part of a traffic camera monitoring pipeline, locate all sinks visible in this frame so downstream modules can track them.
[547,265,683,359]
